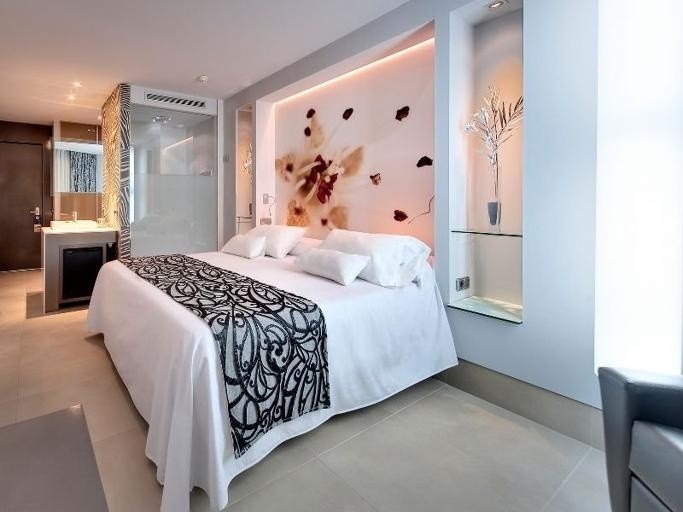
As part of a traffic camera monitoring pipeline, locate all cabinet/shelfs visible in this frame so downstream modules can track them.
[41,221,117,314]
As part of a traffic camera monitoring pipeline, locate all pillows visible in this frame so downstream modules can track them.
[220,224,432,290]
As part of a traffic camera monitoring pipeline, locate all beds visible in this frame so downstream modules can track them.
[82,224,458,512]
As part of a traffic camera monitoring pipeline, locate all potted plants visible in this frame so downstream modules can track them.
[464,82,524,234]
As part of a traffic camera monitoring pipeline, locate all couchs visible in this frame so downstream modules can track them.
[598,365,683,512]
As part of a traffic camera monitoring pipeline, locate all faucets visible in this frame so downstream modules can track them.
[72,212,78,220]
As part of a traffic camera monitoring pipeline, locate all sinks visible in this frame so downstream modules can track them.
[50,220,97,229]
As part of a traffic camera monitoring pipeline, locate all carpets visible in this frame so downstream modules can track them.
[0,403,109,512]
